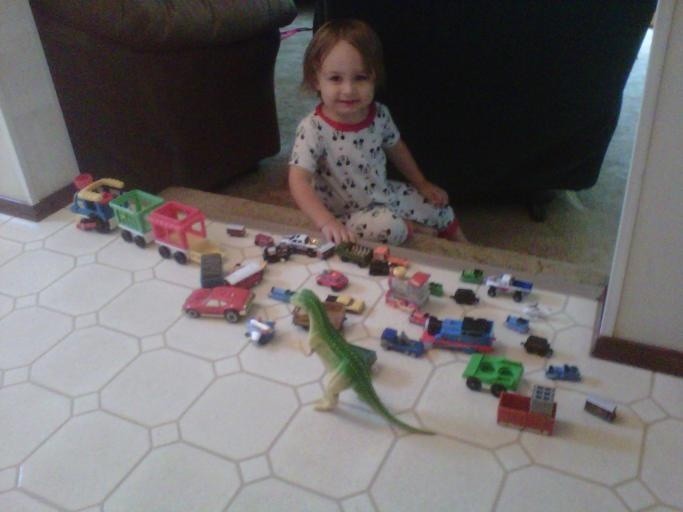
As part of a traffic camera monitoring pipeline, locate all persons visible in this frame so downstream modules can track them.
[285,16,469,249]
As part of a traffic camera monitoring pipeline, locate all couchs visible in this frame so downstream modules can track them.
[30,3,305,189]
[313,0,655,202]
